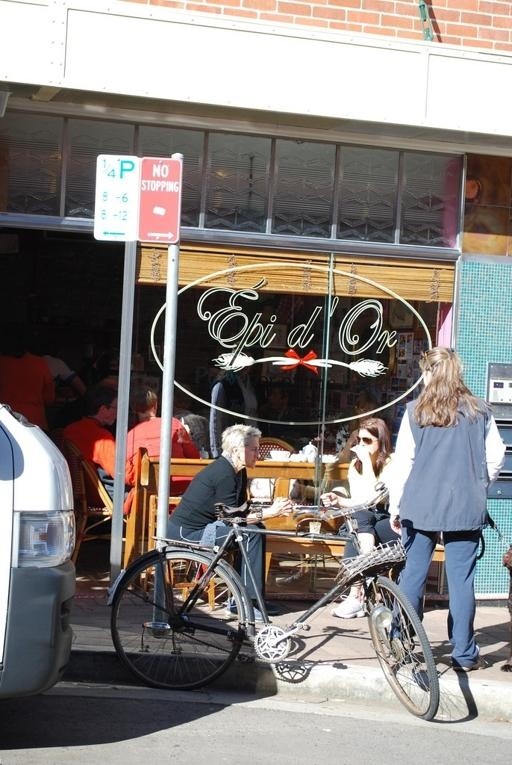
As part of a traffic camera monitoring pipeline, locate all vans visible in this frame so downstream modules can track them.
[0,402,79,699]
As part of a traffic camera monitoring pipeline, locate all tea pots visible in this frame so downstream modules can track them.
[303,441,319,451]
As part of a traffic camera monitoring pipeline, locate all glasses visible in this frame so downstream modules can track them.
[356,436,379,445]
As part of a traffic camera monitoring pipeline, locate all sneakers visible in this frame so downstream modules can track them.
[275,571,310,590]
[331,597,366,619]
[452,657,490,673]
[230,599,281,616]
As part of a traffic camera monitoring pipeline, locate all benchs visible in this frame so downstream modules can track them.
[141,453,449,605]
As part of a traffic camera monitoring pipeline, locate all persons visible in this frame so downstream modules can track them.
[209,342,265,459]
[320,417,402,619]
[389,345,505,672]
[336,391,381,453]
[166,424,282,623]
[1,322,208,514]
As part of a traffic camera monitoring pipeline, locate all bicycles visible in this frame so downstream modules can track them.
[105,482,447,726]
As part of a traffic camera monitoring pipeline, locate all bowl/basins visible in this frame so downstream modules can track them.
[270,450,290,461]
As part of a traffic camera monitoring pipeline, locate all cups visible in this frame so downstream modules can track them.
[291,451,340,463]
[309,522,321,534]
